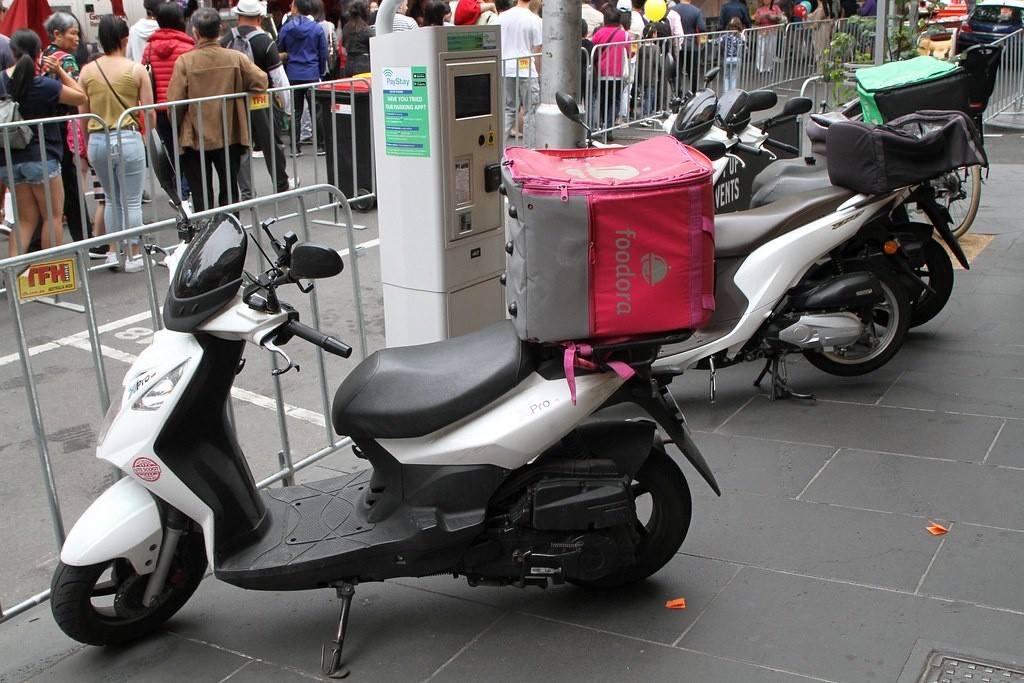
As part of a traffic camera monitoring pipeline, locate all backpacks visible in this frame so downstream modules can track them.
[226,28,266,64]
[0,72,34,150]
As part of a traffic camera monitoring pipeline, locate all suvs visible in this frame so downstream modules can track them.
[955,0,1024,71]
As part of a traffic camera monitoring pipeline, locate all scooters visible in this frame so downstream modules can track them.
[555,54,981,401]
[49,127,721,678]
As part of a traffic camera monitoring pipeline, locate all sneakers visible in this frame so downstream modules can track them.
[288,177,300,191]
[125,254,156,272]
[0,220,15,236]
[105,251,124,268]
[317,146,326,156]
[290,148,302,157]
[88,244,124,258]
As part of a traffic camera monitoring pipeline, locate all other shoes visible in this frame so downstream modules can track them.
[142,190,151,202]
[61,215,67,225]
[169,199,192,218]
[608,131,614,142]
[510,128,523,138]
[640,119,653,126]
[252,144,264,156]
[615,121,629,128]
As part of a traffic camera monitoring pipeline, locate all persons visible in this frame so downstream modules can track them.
[0,0,542,270]
[581,0,877,140]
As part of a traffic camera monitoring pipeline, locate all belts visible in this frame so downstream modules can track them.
[91,126,140,133]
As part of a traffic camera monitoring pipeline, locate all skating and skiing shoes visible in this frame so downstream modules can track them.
[68,118,87,156]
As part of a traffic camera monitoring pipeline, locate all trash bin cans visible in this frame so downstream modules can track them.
[307,72,377,213]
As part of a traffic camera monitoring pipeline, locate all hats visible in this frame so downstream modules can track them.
[617,0,632,12]
[230,0,263,16]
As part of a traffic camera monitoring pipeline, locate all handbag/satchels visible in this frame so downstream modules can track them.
[328,31,340,74]
[623,31,635,85]
[145,64,157,104]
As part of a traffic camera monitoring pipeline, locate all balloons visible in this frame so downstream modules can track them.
[800,1,812,14]
[644,0,667,23]
[794,5,807,18]
